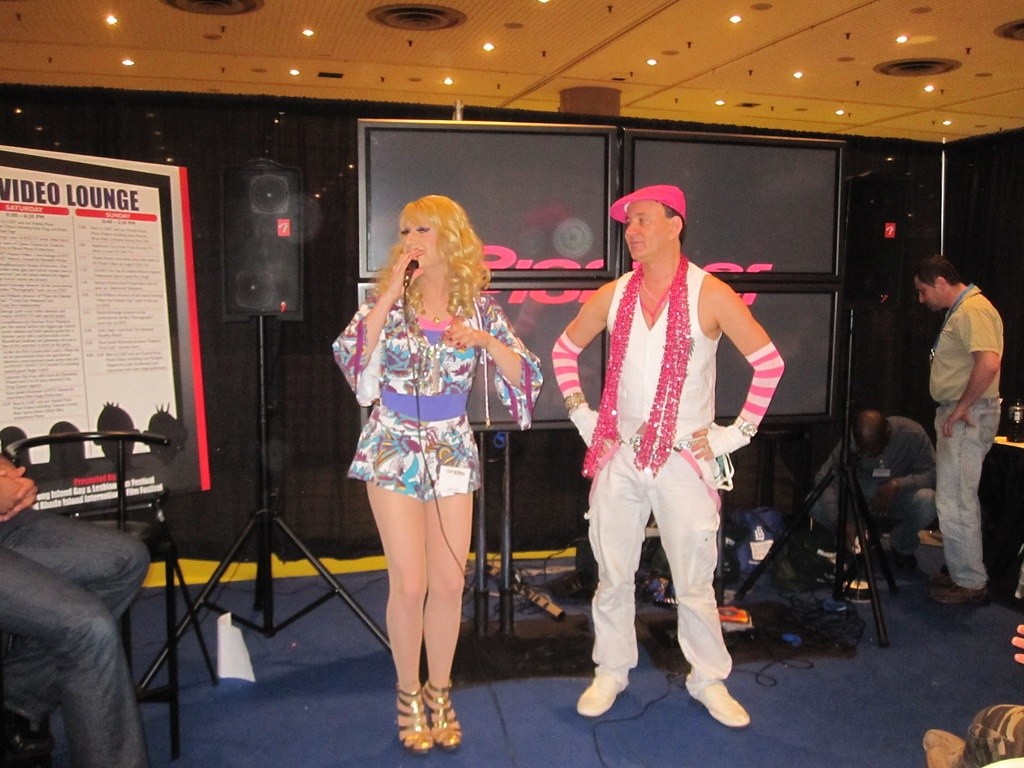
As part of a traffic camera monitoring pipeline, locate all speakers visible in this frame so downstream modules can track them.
[841,168,907,308]
[219,164,304,321]
[572,527,673,600]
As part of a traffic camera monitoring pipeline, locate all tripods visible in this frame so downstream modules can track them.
[733,304,899,646]
[137,319,391,694]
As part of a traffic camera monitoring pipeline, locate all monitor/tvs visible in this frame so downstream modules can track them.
[359,119,847,432]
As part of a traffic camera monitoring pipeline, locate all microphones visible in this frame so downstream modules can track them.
[403,260,419,287]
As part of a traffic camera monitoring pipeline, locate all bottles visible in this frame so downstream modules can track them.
[1007,398,1024,443]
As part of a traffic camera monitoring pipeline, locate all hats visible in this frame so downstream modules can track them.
[610,180,687,225]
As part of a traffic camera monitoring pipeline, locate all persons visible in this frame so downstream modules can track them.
[0,451,151,767]
[809,412,937,569]
[550,183,785,729]
[331,193,548,754]
[959,622,1024,768]
[914,257,1005,606]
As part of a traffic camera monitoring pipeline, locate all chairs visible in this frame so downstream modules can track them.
[5,429,187,758]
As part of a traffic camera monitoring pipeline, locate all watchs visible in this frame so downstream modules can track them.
[735,416,757,437]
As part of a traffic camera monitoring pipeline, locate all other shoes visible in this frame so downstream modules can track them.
[935,581,991,604]
[0,710,53,758]
[922,728,967,768]
[690,682,751,729]
[576,671,627,718]
[891,542,916,572]
[857,552,873,579]
[928,563,955,588]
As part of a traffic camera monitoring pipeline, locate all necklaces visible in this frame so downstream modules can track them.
[581,252,692,476]
[424,298,448,324]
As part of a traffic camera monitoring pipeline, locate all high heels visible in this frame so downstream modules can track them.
[395,679,433,755]
[422,676,462,752]
